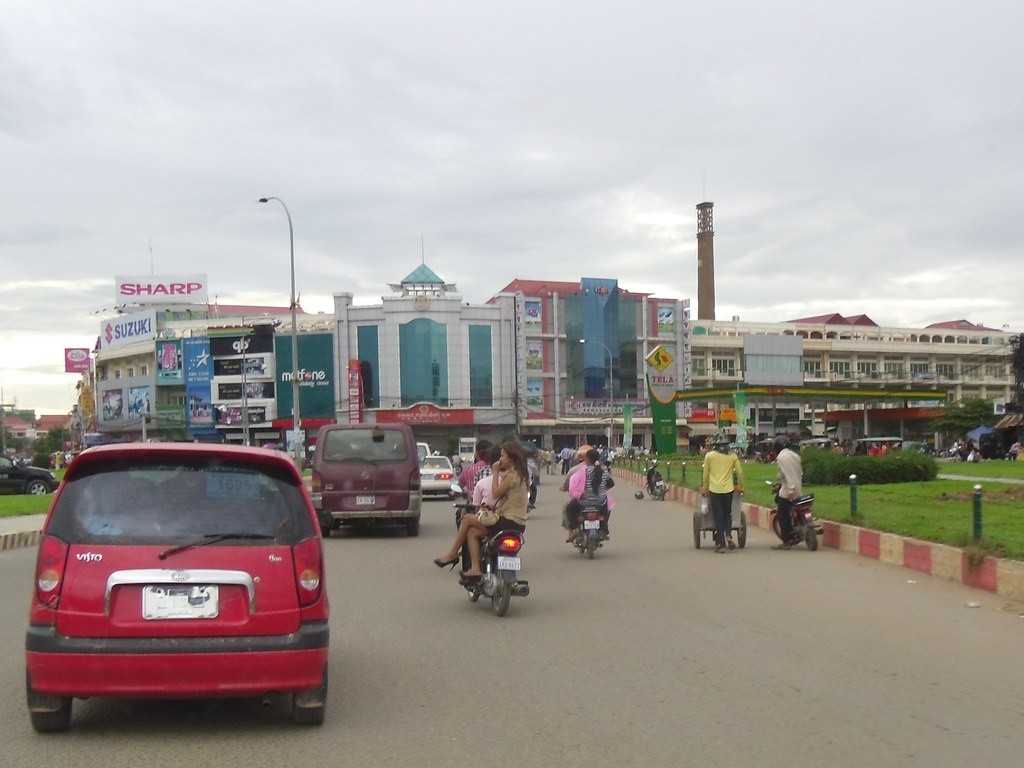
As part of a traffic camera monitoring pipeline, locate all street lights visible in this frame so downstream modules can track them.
[578,339,612,448]
[260,197,302,474]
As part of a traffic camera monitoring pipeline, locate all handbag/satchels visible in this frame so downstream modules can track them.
[476,509,499,526]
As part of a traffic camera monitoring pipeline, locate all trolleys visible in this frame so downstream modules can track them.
[693,492,748,549]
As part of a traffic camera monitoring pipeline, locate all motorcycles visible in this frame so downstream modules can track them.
[559,488,607,559]
[453,457,466,476]
[764,480,823,551]
[643,459,668,501]
[450,484,530,616]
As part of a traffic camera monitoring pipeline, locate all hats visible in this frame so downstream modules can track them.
[710,432,731,444]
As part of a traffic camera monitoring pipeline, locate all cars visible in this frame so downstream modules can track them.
[419,455,461,500]
[23,442,328,730]
[0,454,60,496]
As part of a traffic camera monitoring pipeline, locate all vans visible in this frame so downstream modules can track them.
[311,422,423,537]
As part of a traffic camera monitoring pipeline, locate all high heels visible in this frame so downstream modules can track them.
[459,571,481,586]
[433,557,460,572]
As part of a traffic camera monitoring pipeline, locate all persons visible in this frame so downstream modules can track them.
[434,440,529,583]
[11,437,97,511]
[752,432,1023,465]
[543,436,651,472]
[559,445,610,527]
[473,445,499,487]
[473,444,505,514]
[455,441,493,501]
[698,432,744,553]
[770,436,804,550]
[566,449,615,543]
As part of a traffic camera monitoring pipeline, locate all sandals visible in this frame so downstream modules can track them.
[712,547,725,553]
[728,539,736,550]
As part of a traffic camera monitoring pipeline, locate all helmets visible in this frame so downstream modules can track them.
[774,435,791,452]
[577,445,594,461]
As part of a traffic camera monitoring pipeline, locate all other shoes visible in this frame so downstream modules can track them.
[770,542,790,550]
[566,532,578,543]
[599,530,609,541]
[528,503,535,509]
